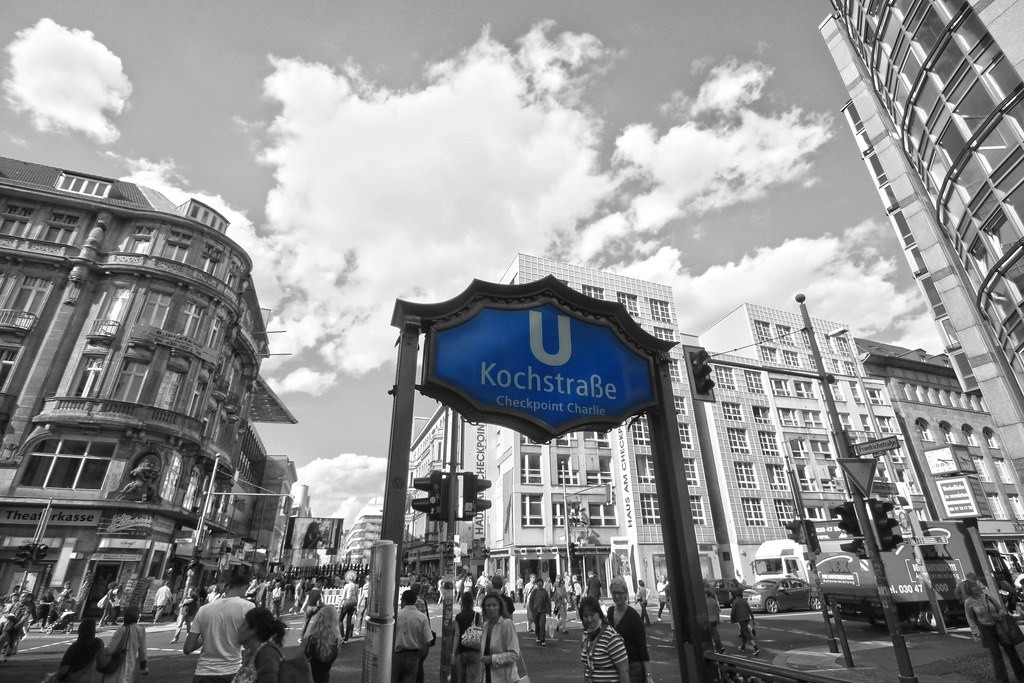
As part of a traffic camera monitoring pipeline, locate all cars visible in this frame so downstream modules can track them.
[1001,552,1024,602]
[707,578,752,608]
[742,577,823,614]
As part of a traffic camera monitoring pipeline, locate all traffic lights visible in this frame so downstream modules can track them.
[32,544,49,563]
[412,469,441,520]
[14,543,32,568]
[784,520,807,544]
[188,545,203,569]
[866,498,904,551]
[833,502,861,535]
[605,482,616,506]
[683,345,716,401]
[460,472,492,521]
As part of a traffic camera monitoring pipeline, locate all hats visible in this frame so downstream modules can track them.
[588,571,595,575]
[344,571,357,583]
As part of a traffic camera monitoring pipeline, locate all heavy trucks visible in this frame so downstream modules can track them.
[807,516,1023,632]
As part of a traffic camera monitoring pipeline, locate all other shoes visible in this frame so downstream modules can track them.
[563,630,568,633]
[112,622,119,625]
[719,648,724,654]
[529,629,535,634]
[738,646,745,652]
[40,629,46,634]
[556,625,559,631]
[99,624,104,627]
[170,638,178,644]
[752,649,761,655]
[536,637,546,646]
[658,617,662,622]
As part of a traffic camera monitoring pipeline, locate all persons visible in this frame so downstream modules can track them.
[963,579,1024,683]
[953,572,987,643]
[0,569,761,683]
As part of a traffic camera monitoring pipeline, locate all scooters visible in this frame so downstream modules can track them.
[45,602,76,635]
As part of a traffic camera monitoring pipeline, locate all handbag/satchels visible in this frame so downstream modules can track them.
[428,631,436,646]
[461,611,483,650]
[996,614,1024,649]
[255,644,315,683]
[514,675,530,683]
[546,617,555,639]
[343,597,356,613]
[97,595,107,609]
[97,625,131,676]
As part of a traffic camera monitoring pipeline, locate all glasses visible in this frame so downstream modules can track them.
[611,590,626,596]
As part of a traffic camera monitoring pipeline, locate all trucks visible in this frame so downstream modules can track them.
[750,538,811,585]
